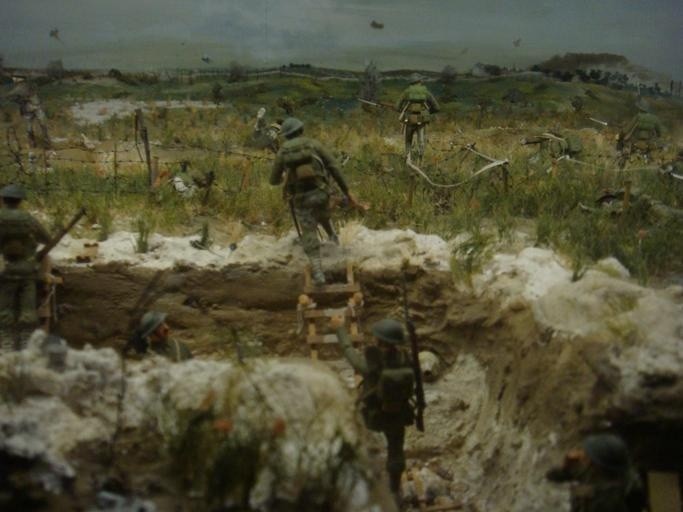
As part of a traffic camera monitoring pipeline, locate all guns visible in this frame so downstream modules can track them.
[266,129,301,239]
[355,97,395,110]
[402,281,424,431]
[135,107,151,186]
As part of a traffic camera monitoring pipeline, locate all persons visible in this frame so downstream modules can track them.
[0,184,53,355]
[393,71,441,163]
[135,305,196,362]
[615,97,664,140]
[545,415,650,512]
[330,312,420,496]
[520,122,581,164]
[578,182,683,227]
[270,116,371,288]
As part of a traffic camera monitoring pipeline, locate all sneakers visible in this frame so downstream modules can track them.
[313,277,327,285]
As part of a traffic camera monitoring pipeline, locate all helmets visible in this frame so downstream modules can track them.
[409,72,423,84]
[281,117,305,137]
[0,184,26,200]
[587,435,624,479]
[140,310,168,339]
[374,318,405,344]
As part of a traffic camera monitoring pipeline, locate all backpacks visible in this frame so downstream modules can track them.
[379,360,416,420]
[283,140,328,209]
[407,91,431,125]
